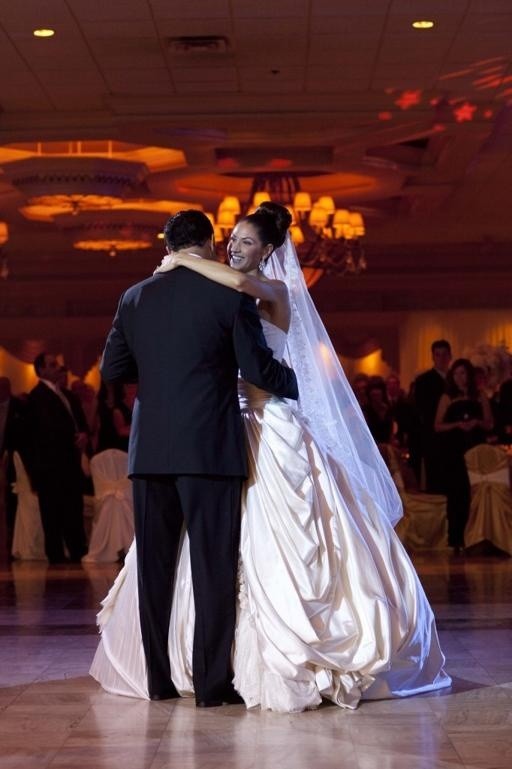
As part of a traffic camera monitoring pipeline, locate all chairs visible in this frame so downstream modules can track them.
[376,440,512,558]
[0,445,137,564]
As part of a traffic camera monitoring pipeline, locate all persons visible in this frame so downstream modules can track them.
[152,204,389,712]
[97,210,299,706]
[354,336,511,555]
[0,334,139,567]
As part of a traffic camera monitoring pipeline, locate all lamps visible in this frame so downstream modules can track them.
[202,171,369,291]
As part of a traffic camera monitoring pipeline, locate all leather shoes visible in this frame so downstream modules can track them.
[149,680,182,702]
[193,686,246,708]
